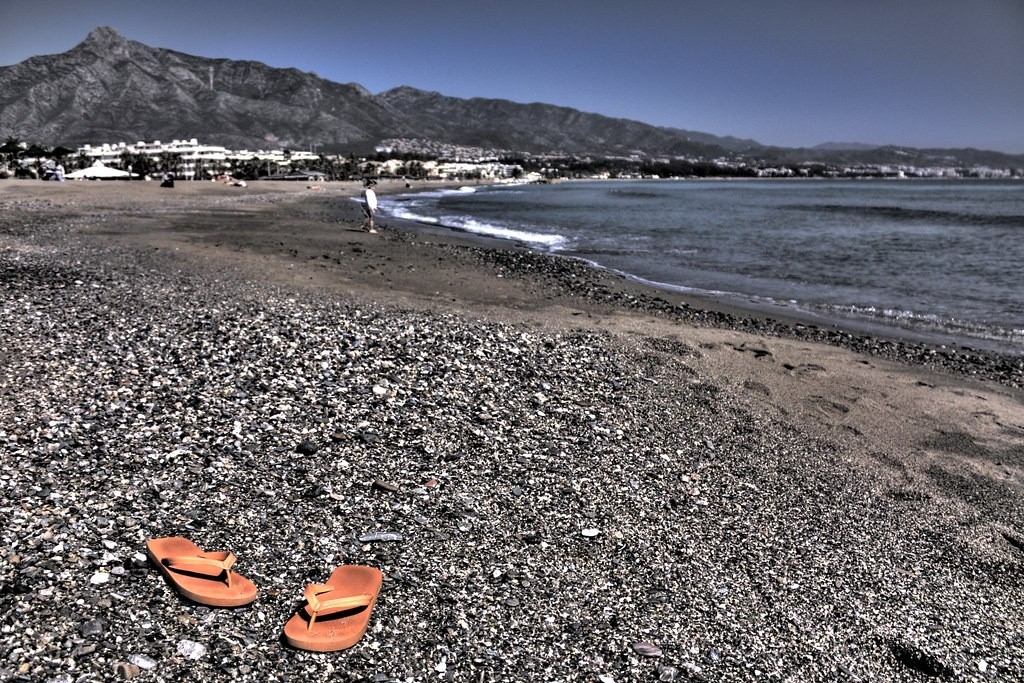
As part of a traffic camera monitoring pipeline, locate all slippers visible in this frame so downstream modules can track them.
[148,537,257,606]
[285,564,382,652]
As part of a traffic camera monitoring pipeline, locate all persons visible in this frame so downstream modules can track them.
[360,180,379,233]
[127,163,133,180]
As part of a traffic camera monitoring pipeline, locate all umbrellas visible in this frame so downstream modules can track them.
[45,146,75,165]
[19,144,48,162]
[0,144,24,169]
